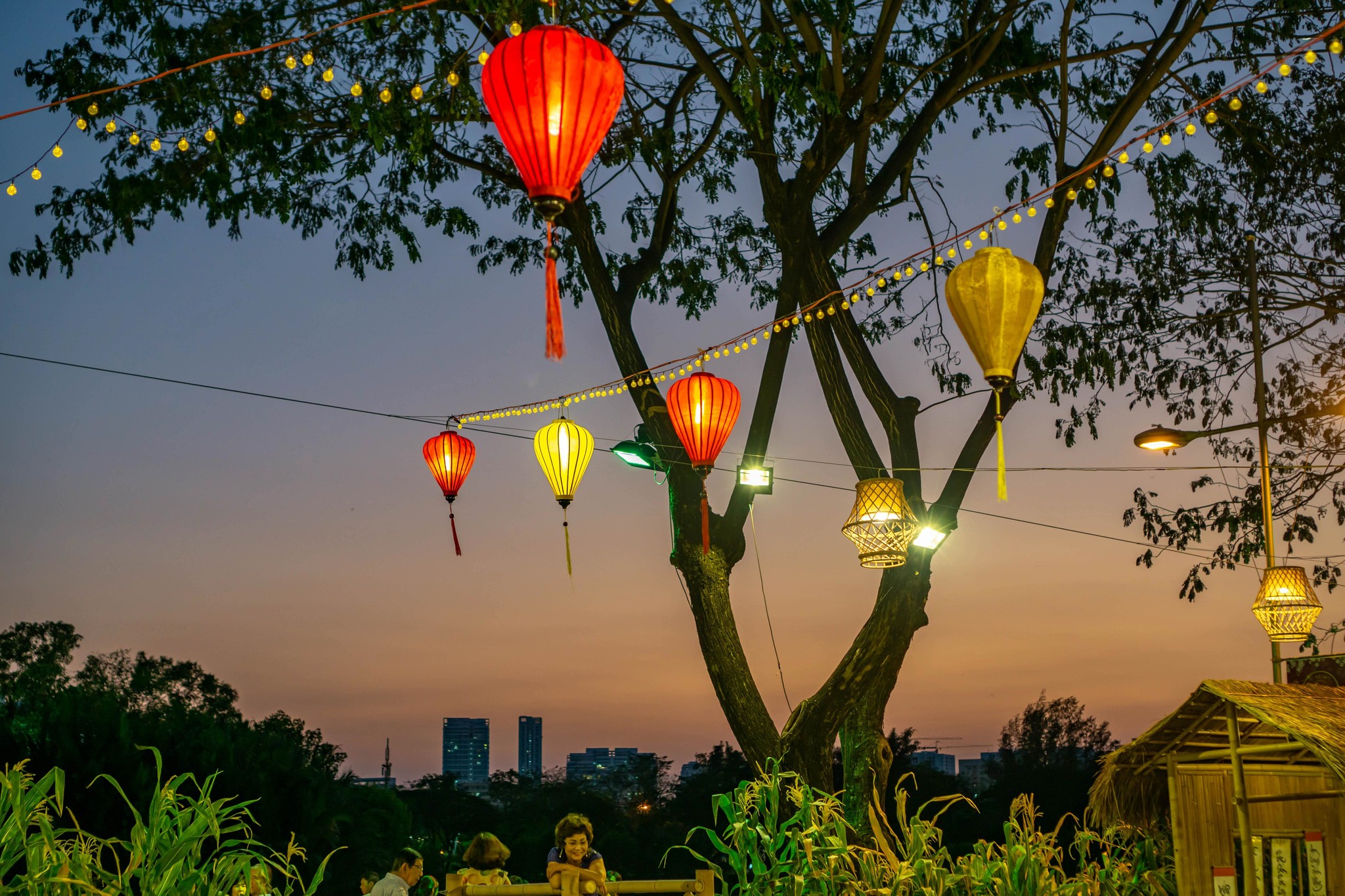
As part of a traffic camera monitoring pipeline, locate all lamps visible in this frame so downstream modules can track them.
[609,423,667,472]
[912,520,948,551]
[736,465,774,495]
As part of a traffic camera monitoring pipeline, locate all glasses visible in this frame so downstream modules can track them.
[563,840,589,846]
[413,863,424,873]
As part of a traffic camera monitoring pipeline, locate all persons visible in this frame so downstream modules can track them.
[462,832,511,886]
[545,810,608,896]
[224,864,272,896]
[370,848,424,896]
[360,871,380,896]
[412,875,439,896]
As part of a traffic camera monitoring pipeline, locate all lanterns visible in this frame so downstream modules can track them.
[840,475,923,569]
[668,373,741,554]
[948,242,1045,496]
[534,420,591,575]
[423,428,477,557]
[1251,561,1322,643]
[482,22,624,359]
